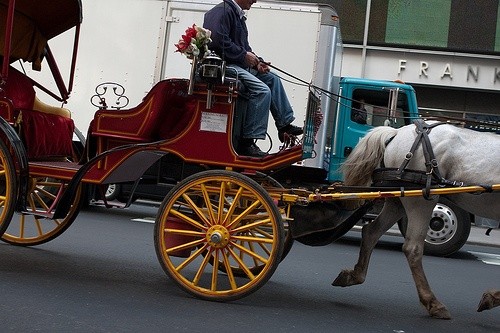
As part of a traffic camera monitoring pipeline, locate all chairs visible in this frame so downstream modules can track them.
[188,56,246,97]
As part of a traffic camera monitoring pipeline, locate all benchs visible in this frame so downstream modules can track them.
[90,78,190,142]
[0,66,74,158]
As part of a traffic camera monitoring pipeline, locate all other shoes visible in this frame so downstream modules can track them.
[234,142,269,157]
[278,124,304,143]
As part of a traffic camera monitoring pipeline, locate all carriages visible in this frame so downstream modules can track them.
[0,0,500,319]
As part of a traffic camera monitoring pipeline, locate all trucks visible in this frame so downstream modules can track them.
[9,0,471,255]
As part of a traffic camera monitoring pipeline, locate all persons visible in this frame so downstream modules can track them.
[352,95,367,124]
[202,0,305,158]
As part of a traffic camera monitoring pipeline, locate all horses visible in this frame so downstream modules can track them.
[331,119,500,319]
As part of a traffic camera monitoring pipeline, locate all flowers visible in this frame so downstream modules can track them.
[174,23,213,61]
